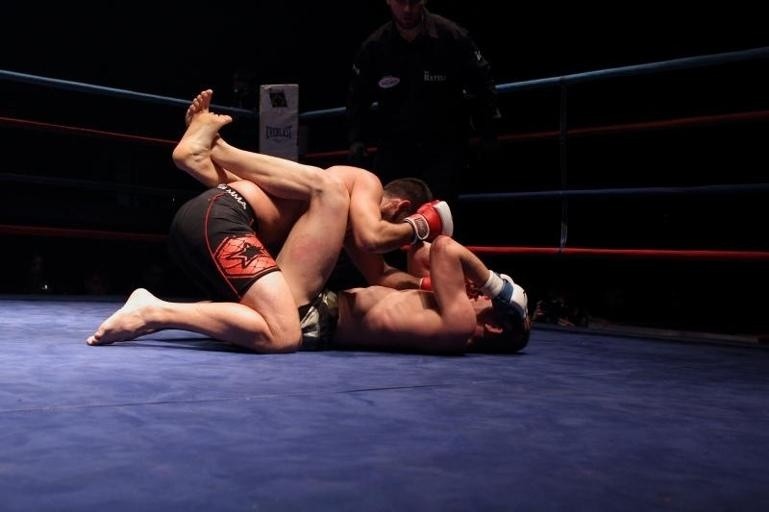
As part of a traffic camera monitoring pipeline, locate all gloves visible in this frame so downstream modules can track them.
[420,276,434,292]
[407,198,454,245]
[491,271,531,320]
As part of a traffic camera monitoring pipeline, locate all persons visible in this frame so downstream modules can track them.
[531,283,610,331]
[345,0,497,201]
[169,88,534,355]
[83,162,456,352]
